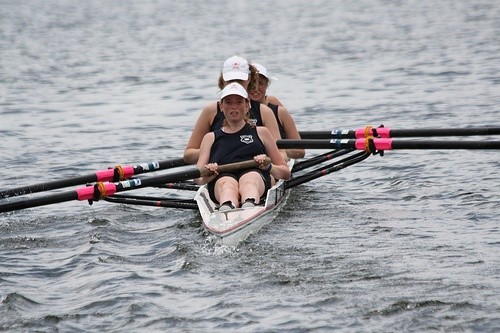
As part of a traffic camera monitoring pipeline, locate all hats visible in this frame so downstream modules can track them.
[220,82,248,99]
[222,56,248,81]
[251,63,271,81]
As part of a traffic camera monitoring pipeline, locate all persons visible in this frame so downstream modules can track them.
[184,55,306,164]
[194,83,291,212]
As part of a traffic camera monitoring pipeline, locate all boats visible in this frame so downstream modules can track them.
[193,156,295,246]
